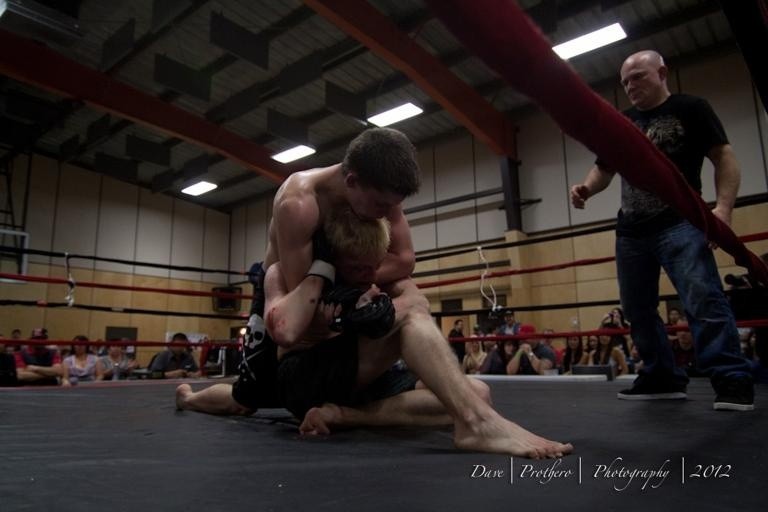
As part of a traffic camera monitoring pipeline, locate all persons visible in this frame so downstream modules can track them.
[0,125,768,461]
[570,49,757,412]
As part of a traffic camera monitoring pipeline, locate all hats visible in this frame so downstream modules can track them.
[517,325,537,338]
[32,328,49,339]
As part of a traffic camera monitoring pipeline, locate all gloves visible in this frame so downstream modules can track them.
[307,226,339,285]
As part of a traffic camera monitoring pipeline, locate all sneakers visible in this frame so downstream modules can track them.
[616,377,690,402]
[713,387,754,411]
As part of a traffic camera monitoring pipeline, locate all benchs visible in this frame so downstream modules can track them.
[120,369,150,380]
